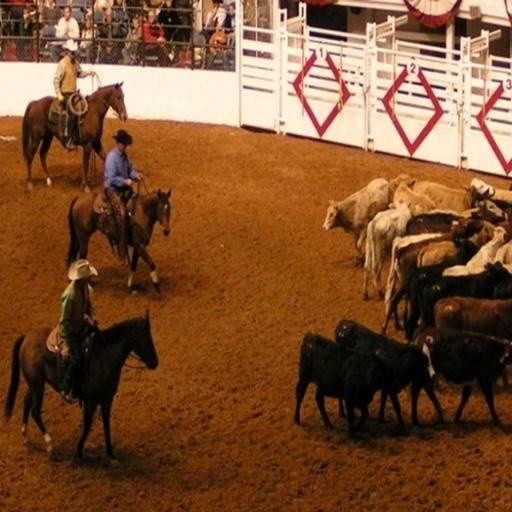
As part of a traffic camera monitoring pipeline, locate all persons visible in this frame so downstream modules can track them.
[53,39,95,151]
[103,129,144,264]
[0,0,236,73]
[57,259,99,404]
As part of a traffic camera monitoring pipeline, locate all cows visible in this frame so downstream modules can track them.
[321,175,509,379]
[293,334,400,435]
[332,317,435,435]
[411,331,512,436]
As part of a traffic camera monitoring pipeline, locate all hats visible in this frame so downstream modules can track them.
[112,129,132,145]
[68,259,98,280]
[62,39,78,51]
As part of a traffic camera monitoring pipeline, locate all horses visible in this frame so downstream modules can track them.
[23,80,127,193]
[65,188,171,300]
[2,308,158,469]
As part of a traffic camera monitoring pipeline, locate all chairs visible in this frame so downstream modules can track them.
[0,1,235,66]
